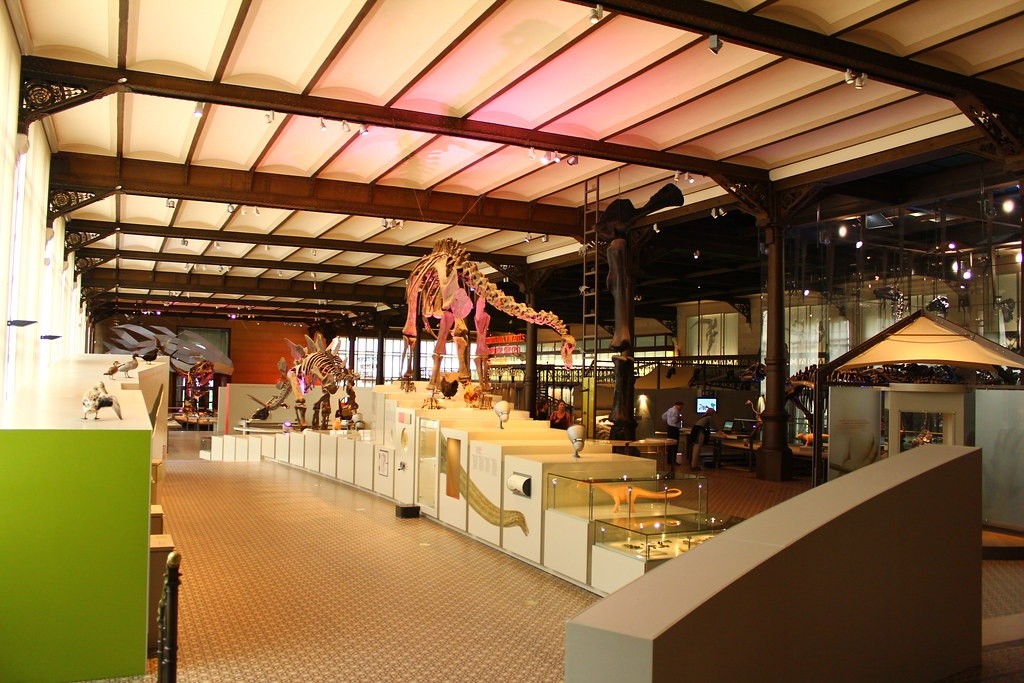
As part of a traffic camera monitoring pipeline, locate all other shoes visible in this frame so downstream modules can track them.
[670,461,681,466]
[691,465,701,471]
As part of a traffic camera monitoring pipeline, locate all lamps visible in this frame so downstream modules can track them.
[320,118,327,131]
[844,68,868,89]
[567,424,587,458]
[181,237,188,246]
[264,110,275,124]
[711,207,727,218]
[359,124,368,136]
[351,412,364,431]
[166,197,175,209]
[184,263,231,273]
[708,34,724,56]
[342,119,351,133]
[241,204,260,216]
[381,217,404,230]
[674,170,681,181]
[590,4,603,24]
[214,240,221,249]
[525,231,549,243]
[493,400,510,429]
[653,223,660,234]
[685,172,694,183]
[529,146,579,166]
[193,101,204,118]
[227,203,235,214]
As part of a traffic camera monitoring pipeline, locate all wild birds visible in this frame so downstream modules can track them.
[118,353,142,378]
[103,361,121,380]
[81,381,124,420]
[141,349,159,365]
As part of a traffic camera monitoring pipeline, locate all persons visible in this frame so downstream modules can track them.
[550,400,570,430]
[690,408,727,471]
[662,402,684,466]
[537,399,550,420]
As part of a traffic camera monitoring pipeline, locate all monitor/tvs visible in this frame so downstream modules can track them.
[695,397,719,414]
[723,419,735,431]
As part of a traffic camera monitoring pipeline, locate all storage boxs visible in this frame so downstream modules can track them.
[396,504,420,519]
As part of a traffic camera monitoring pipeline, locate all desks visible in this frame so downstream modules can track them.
[601,427,828,482]
